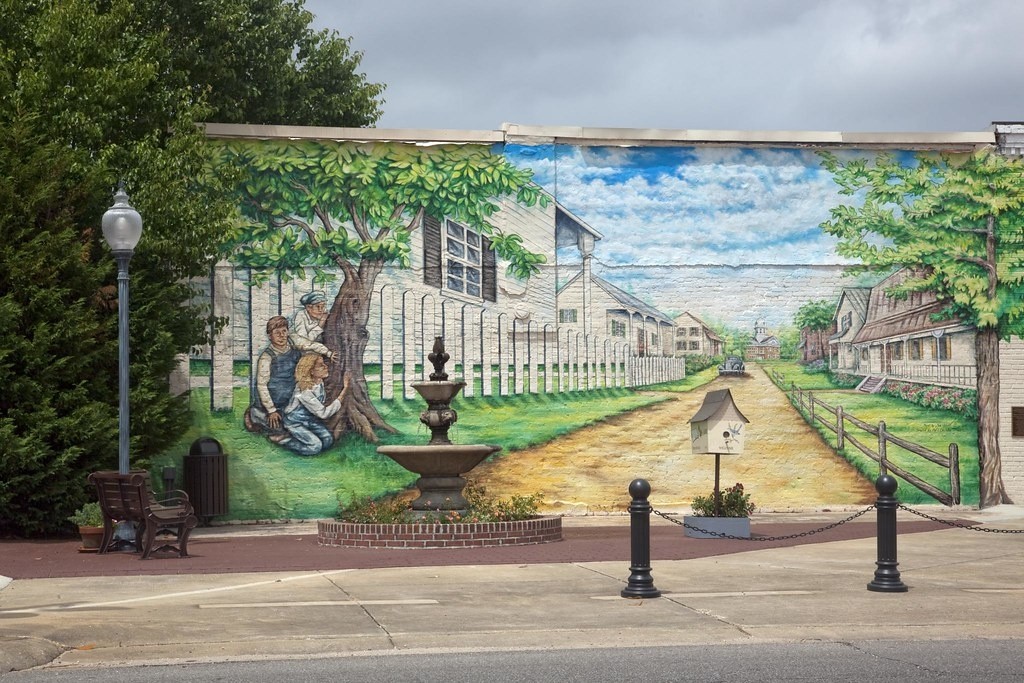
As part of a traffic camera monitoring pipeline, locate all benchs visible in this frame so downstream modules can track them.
[87,466,197,560]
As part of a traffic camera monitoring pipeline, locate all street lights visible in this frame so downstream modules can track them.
[102,179,143,551]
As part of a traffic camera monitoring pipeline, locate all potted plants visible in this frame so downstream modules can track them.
[66,500,118,548]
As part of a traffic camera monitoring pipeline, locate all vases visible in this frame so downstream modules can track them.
[683,515,751,540]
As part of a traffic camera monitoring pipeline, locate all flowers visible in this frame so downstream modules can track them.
[690,482,756,517]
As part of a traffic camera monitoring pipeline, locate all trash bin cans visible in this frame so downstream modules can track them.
[181,435,229,526]
